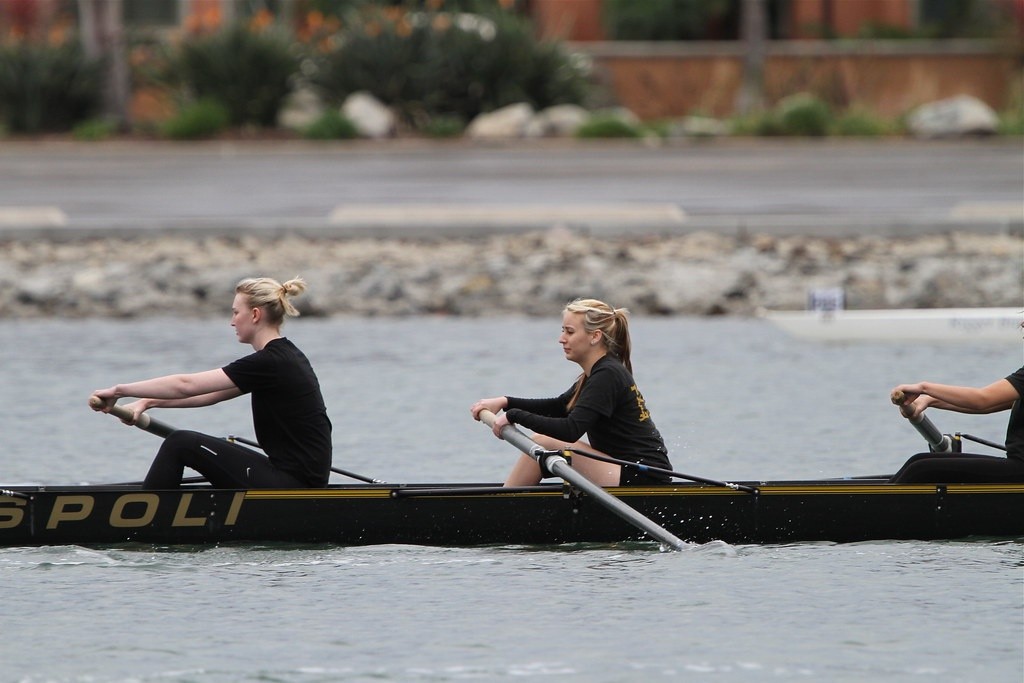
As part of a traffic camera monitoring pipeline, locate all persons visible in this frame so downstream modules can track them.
[88,277,333,489]
[890,365,1024,484]
[472,299,672,487]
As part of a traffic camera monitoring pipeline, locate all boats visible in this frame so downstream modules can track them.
[0,472,1024,551]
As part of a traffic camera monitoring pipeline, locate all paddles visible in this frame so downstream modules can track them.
[477,408,693,554]
[893,388,952,455]
[92,398,177,440]
